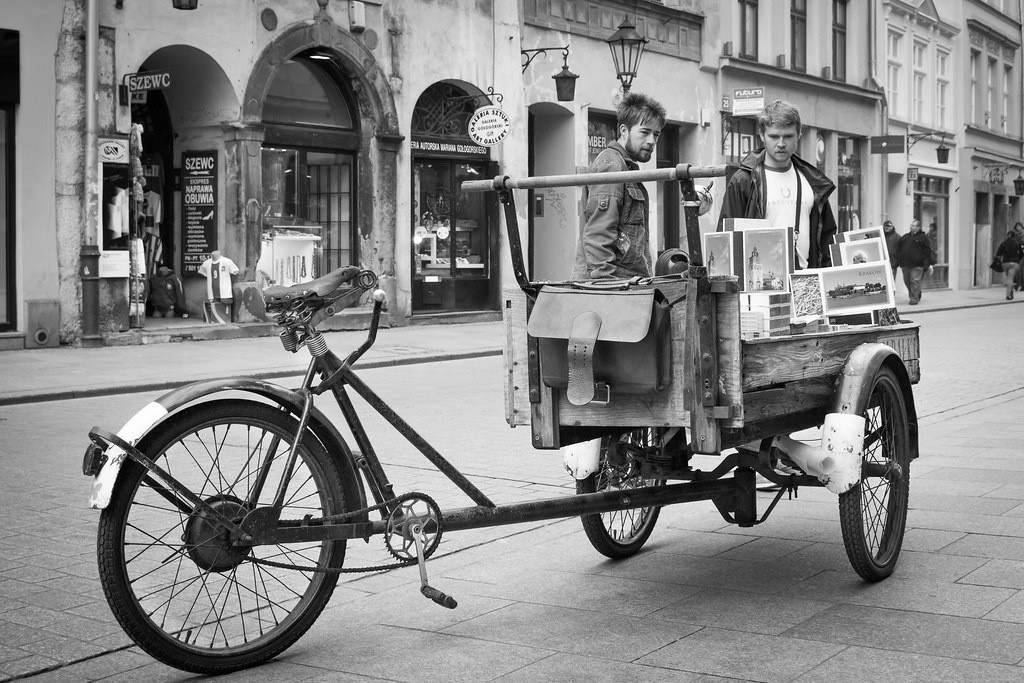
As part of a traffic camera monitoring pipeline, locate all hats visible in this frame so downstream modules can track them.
[1007,230,1018,238]
[883,221,893,227]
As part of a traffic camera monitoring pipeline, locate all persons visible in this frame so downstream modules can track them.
[1013,223,1024,291]
[996,231,1024,300]
[927,223,937,257]
[572,93,666,492]
[883,221,900,280]
[716,100,837,488]
[198,251,239,299]
[150,267,184,318]
[900,220,936,305]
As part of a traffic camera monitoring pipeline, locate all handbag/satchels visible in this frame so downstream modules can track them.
[989,256,1003,273]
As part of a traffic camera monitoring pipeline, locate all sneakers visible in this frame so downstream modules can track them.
[738,450,805,491]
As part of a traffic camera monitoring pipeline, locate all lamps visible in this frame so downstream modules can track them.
[985,163,1024,196]
[521,44,580,101]
[906,127,950,163]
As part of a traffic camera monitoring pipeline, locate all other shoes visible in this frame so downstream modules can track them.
[1013,284,1018,291]
[1006,296,1013,300]
[908,298,922,305]
[1020,286,1024,291]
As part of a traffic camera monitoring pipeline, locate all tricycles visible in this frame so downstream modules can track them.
[82,163,921,677]
